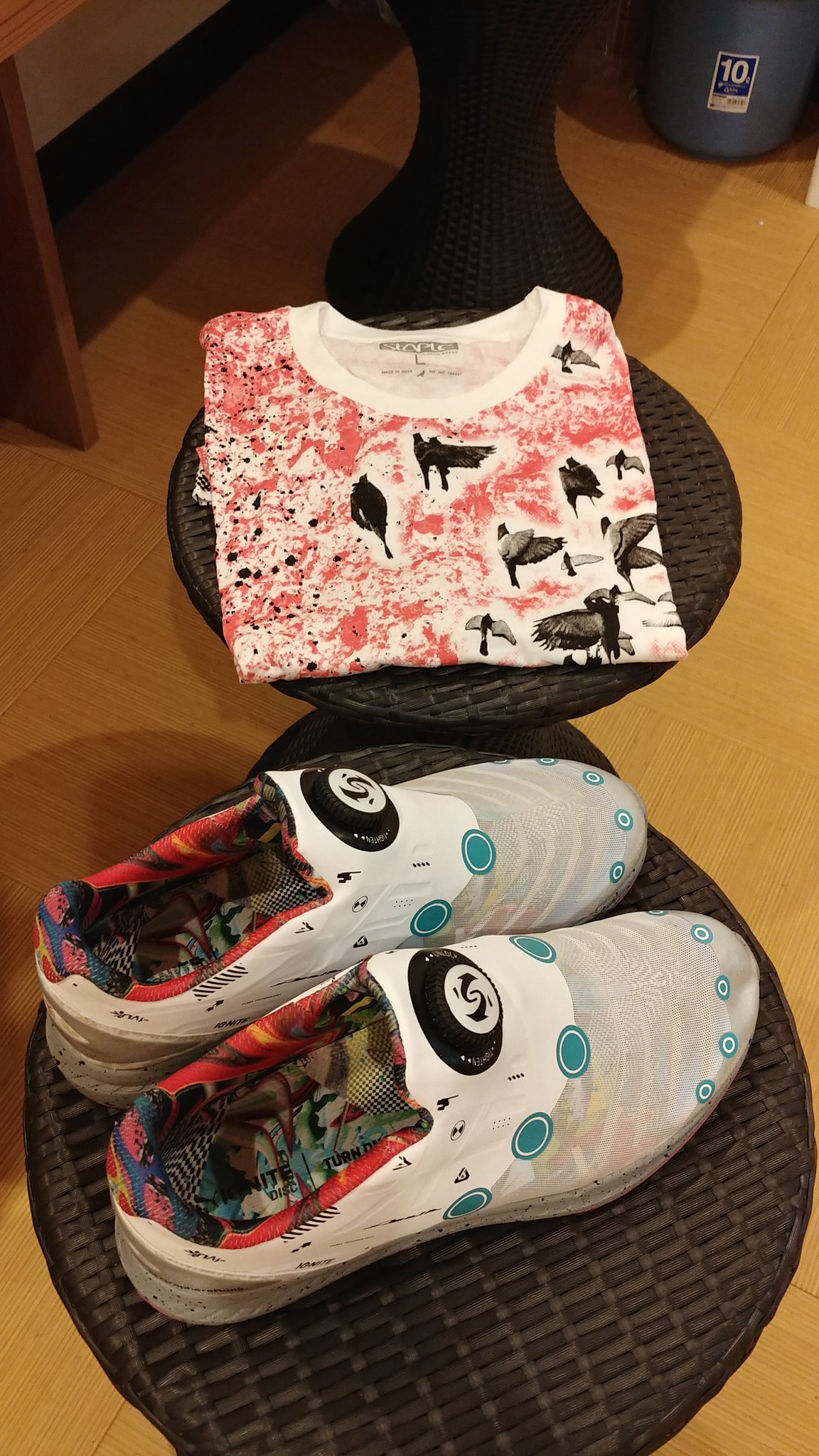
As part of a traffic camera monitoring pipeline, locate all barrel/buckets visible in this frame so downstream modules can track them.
[633,1,819,157]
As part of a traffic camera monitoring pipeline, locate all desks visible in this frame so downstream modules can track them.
[15,2,819,1456]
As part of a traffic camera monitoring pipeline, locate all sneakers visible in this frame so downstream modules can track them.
[101,909,760,1325]
[34,756,649,1112]
